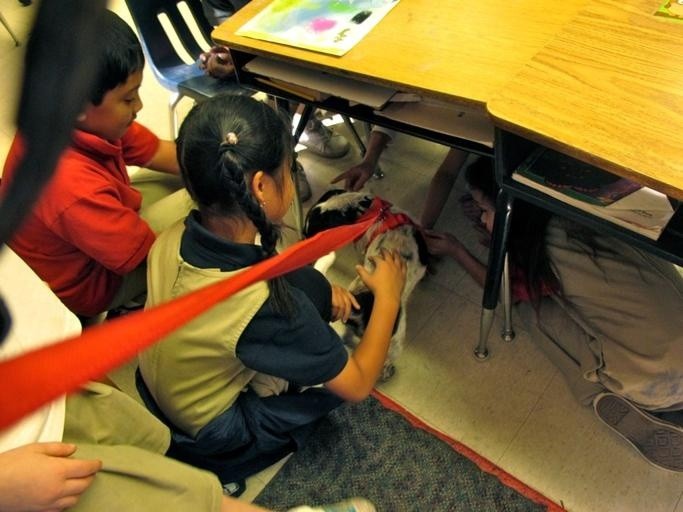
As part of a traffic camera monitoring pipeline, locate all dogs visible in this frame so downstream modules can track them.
[303,187,431,385]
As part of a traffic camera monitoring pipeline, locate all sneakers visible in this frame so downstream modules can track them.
[289,151,311,203]
[591,391,683,476]
[291,110,350,159]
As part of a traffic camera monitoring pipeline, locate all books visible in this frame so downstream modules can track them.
[509,143,680,245]
[238,54,423,113]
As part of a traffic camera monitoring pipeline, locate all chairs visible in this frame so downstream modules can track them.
[125,0,260,141]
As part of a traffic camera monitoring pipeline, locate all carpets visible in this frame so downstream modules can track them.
[249,387,570,512]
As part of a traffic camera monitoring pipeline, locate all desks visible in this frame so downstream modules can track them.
[212,0,683,360]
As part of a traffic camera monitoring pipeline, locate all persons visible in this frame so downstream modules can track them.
[422,153,682,472]
[0,2,199,320]
[330,122,397,192]
[136,93,410,483]
[194,0,351,205]
[410,146,482,232]
[0,240,379,511]
